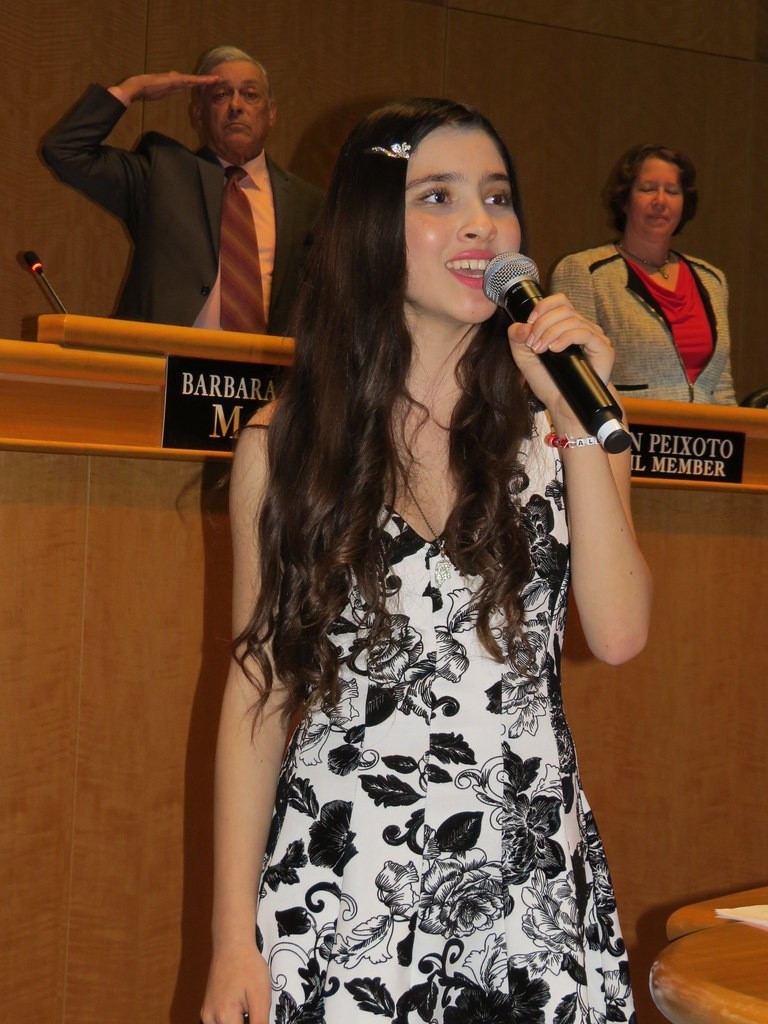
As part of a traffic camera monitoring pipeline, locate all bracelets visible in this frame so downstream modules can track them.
[545,433,599,449]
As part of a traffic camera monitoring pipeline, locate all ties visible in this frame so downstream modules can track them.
[220,165,266,334]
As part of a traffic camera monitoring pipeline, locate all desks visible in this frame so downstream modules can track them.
[650,886,768,1024]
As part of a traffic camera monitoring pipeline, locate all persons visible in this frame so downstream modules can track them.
[42,46,326,334]
[201,96,651,1024]
[550,143,737,406]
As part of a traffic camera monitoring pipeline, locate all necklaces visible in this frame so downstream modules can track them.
[621,242,671,279]
[400,465,453,586]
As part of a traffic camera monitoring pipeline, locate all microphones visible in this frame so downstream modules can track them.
[25,251,68,315]
[482,252,632,454]
